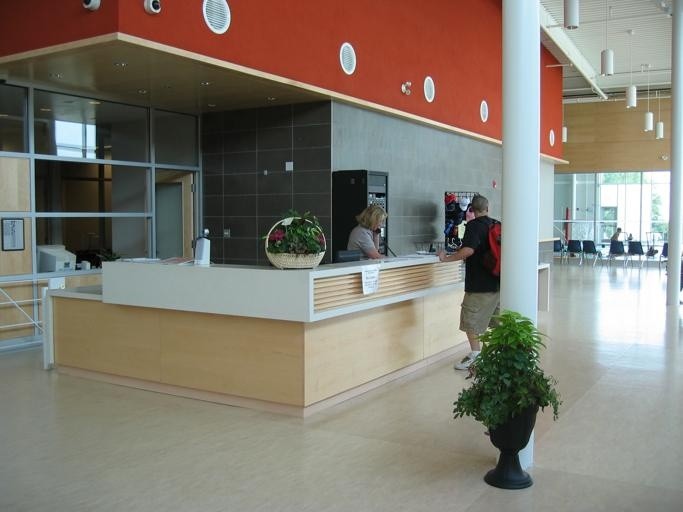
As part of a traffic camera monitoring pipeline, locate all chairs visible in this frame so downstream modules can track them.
[553,238,644,271]
[658,242,667,269]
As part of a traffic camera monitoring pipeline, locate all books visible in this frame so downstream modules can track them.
[156,256,193,265]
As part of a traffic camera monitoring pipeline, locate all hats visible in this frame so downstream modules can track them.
[466,206,475,222]
[454,211,465,225]
[445,194,456,212]
[459,197,471,211]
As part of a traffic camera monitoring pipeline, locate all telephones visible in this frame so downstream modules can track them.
[375,228,380,234]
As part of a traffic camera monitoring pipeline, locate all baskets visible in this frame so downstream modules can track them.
[265,217,326,269]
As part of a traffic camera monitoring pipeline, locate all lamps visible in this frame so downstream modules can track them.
[561,1,666,144]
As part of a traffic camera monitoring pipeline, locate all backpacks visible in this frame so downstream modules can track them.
[476,218,501,275]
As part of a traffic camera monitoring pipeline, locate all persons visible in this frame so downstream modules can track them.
[346,203,389,259]
[433,194,501,371]
[610,227,621,241]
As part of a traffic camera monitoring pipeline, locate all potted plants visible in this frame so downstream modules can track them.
[450,308,563,491]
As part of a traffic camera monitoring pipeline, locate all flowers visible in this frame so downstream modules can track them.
[261,207,325,260]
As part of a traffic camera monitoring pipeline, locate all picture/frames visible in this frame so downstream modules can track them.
[0,218,25,251]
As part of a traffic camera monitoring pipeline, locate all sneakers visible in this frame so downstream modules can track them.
[456,352,483,369]
